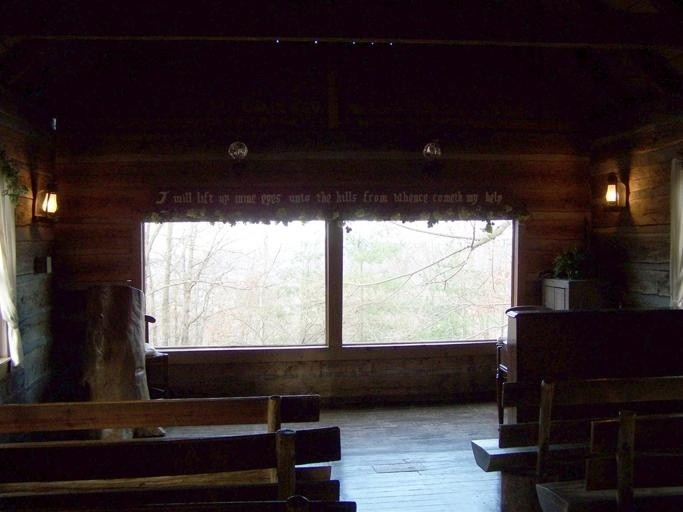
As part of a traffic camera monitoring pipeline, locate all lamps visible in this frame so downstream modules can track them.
[31,169,59,227]
[600,171,630,211]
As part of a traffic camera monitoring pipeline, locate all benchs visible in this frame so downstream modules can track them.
[468,367,682,507]
[1,387,360,512]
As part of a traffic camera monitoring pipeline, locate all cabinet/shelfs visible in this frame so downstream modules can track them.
[541,277,622,313]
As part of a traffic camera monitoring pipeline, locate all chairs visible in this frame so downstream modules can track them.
[141,311,172,399]
